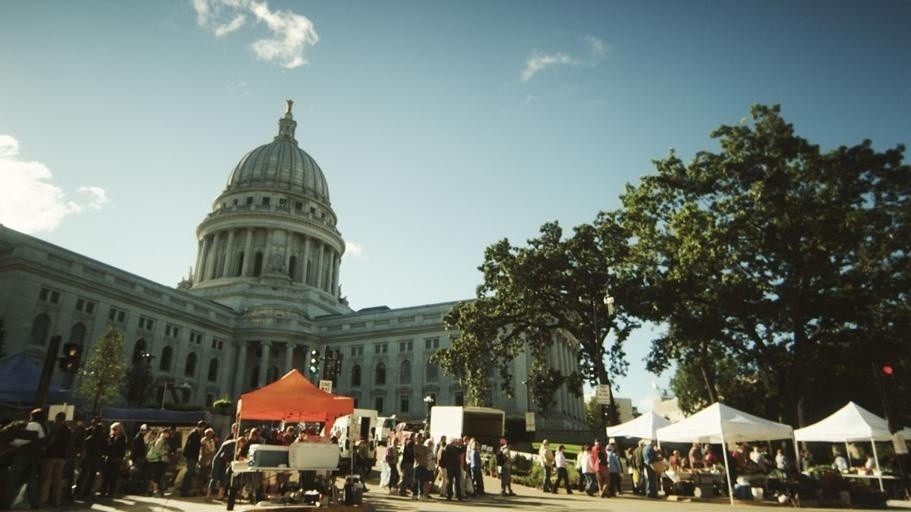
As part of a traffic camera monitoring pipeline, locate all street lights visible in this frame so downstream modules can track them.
[159,382,191,411]
[422,396,434,422]
[592,290,615,445]
[137,351,156,409]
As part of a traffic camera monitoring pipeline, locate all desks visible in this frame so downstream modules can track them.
[660,469,723,496]
[227,467,340,510]
[842,473,899,487]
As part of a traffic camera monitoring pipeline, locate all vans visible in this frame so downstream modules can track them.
[377,414,400,447]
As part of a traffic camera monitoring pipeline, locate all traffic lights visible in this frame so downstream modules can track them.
[875,341,899,380]
[60,342,82,374]
[309,349,318,374]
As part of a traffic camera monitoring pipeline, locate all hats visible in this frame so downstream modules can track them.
[305,426,316,435]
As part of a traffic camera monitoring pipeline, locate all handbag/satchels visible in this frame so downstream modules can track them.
[598,452,609,464]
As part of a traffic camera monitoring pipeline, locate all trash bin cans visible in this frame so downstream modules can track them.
[344,482,363,504]
[696,469,715,498]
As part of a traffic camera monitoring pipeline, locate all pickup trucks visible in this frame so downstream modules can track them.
[387,421,426,449]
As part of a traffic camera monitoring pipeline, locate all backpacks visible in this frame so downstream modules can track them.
[0,420,26,443]
[383,447,393,462]
[146,438,171,463]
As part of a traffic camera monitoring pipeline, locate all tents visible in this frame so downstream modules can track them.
[235,368,354,504]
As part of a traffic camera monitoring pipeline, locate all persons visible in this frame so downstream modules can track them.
[863,450,877,489]
[352,423,516,501]
[574,438,817,499]
[0,406,178,509]
[179,419,342,504]
[830,445,848,475]
[538,439,554,492]
[549,444,573,494]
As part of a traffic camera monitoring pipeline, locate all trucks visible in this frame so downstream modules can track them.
[318,409,378,452]
[421,405,506,459]
